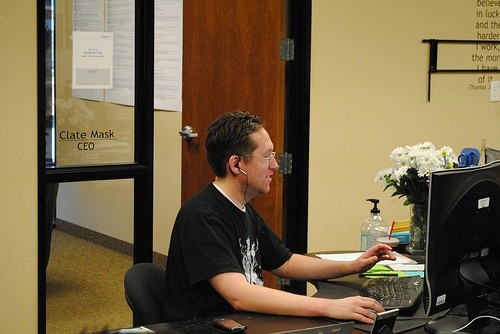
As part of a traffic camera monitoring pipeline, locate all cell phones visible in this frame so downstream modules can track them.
[212,317,247,332]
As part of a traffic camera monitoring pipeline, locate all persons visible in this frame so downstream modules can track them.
[166,111,396,325]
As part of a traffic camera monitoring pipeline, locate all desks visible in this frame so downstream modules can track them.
[103,248,427,334]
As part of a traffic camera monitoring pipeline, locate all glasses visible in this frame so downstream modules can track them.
[239,152,276,164]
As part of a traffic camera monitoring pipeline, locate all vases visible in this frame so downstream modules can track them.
[408,204,427,262]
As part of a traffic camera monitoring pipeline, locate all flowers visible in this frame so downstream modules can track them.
[374,142,459,248]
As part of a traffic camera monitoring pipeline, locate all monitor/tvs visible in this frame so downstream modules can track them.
[423,162,500,334]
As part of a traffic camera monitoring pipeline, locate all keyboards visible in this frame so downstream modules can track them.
[361,274,425,315]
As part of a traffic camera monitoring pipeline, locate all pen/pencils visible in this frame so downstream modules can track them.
[358,273,398,277]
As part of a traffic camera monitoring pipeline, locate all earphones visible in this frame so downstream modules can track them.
[234,163,247,175]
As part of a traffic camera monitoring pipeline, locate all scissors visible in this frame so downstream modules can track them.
[457,152,479,168]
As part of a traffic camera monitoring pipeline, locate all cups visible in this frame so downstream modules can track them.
[377,237,400,252]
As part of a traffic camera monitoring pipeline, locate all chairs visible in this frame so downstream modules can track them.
[125,263,168,325]
